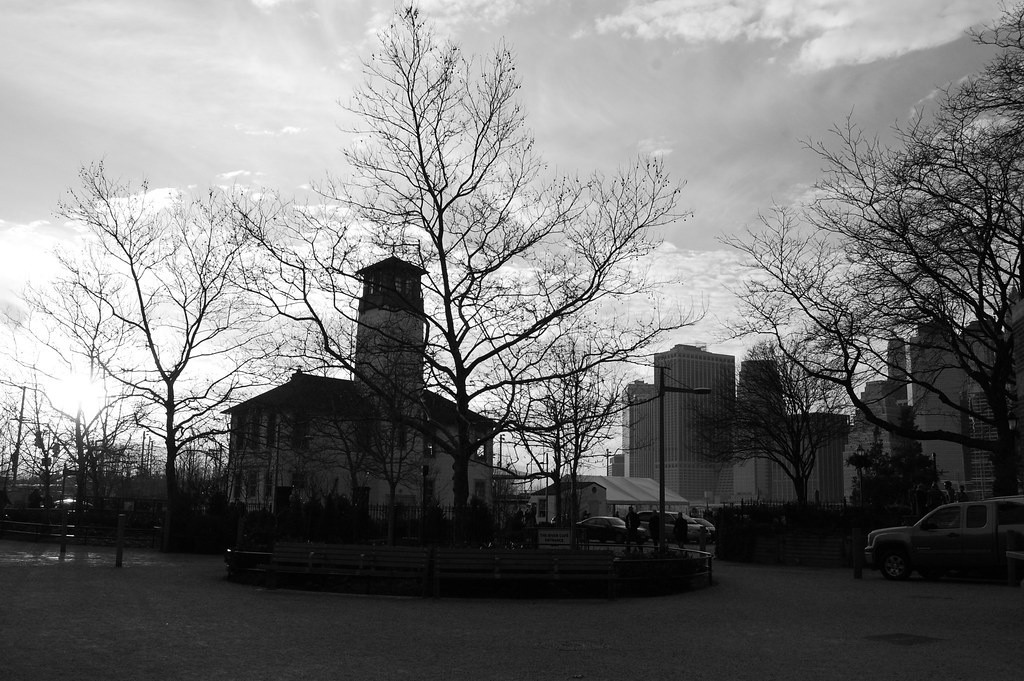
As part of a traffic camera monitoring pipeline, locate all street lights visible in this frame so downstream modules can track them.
[657,384,713,536]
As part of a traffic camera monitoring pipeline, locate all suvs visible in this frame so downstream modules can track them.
[862,495,1024,584]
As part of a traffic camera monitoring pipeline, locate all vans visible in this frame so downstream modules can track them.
[632,510,711,545]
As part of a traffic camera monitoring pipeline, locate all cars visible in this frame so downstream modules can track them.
[575,516,650,545]
[691,517,716,533]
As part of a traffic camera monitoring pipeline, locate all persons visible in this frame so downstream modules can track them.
[625,507,643,552]
[943,480,954,504]
[649,509,660,552]
[957,485,969,502]
[514,507,538,523]
[673,513,688,548]
[615,511,620,518]
[583,510,589,520]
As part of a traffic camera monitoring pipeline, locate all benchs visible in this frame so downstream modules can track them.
[254,541,618,603]
